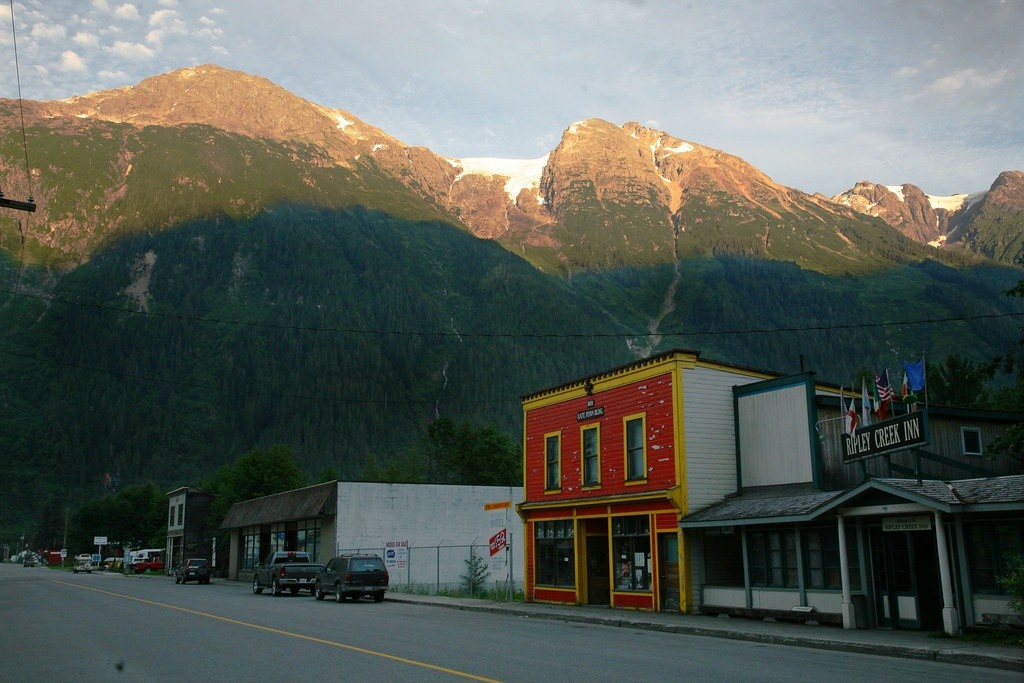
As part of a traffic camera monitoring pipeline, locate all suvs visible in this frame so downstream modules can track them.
[174,558,211,585]
[315,553,390,603]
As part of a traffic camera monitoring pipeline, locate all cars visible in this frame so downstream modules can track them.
[73,557,93,573]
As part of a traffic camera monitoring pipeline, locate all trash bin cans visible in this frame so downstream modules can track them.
[842,594,867,629]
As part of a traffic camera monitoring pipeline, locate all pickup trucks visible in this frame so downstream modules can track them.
[253,551,326,597]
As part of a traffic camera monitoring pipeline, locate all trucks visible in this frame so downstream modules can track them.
[126,557,165,574]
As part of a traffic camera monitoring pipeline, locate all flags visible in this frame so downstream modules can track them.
[839,357,926,438]
